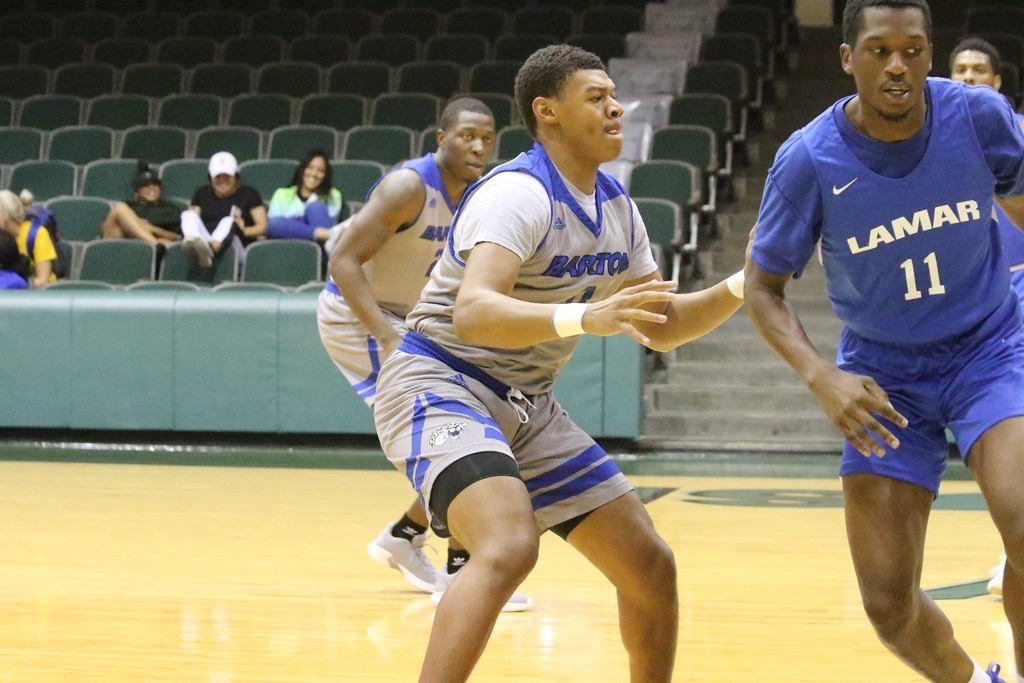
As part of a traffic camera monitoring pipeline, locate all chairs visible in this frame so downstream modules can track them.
[0,0,803,295]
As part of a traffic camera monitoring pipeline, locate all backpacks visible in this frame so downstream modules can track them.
[25,206,61,261]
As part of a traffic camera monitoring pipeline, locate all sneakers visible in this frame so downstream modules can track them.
[987,546,1007,593]
[985,660,1007,683]
[434,565,531,612]
[369,522,437,594]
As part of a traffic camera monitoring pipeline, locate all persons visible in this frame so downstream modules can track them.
[373,44,755,683]
[0,188,59,291]
[101,150,343,272]
[316,96,534,612]
[743,0,1024,683]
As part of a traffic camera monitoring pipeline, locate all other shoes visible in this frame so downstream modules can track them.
[182,235,213,269]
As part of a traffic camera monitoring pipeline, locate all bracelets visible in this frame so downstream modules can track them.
[727,269,744,299]
[552,303,588,338]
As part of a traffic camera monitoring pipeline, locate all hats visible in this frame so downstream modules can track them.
[208,150,239,180]
[132,169,162,192]
